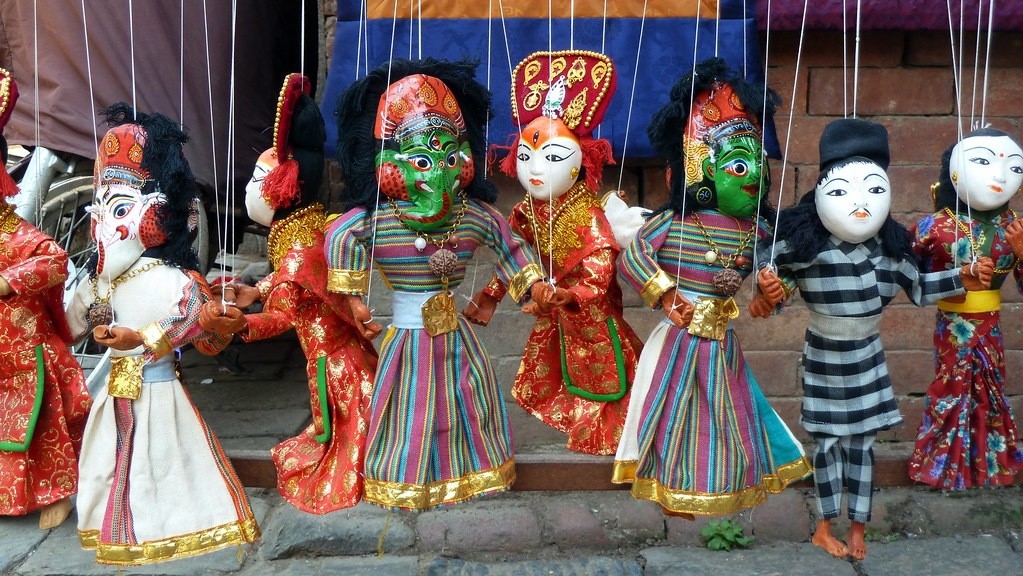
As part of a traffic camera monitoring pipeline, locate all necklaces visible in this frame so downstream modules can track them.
[0,203,17,223]
[944,204,1021,273]
[384,190,467,338]
[87,255,176,329]
[525,187,589,254]
[691,209,758,296]
[267,202,321,261]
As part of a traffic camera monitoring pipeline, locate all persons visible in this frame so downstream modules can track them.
[66,101,262,567]
[197,72,381,515]
[322,56,553,511]
[0,67,93,532]
[755,119,995,559]
[461,50,657,455]
[612,57,812,521]
[906,128,1023,492]
